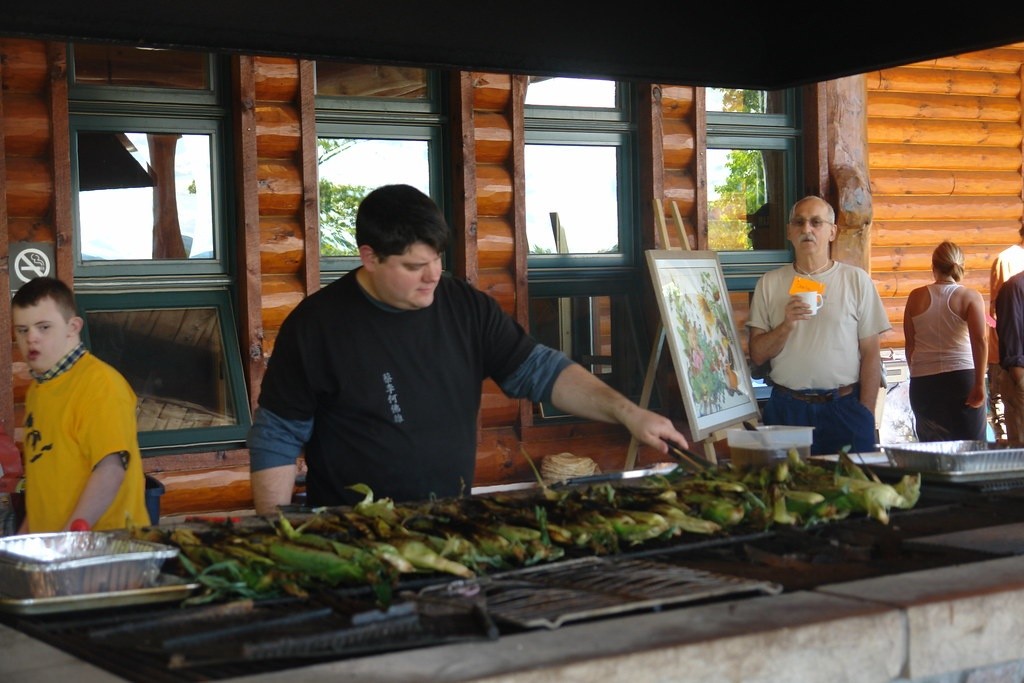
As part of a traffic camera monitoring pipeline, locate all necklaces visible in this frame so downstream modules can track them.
[937,280,955,282]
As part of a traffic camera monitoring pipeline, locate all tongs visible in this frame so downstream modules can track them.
[662,437,715,473]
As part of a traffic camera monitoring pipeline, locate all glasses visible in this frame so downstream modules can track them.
[790,216,835,228]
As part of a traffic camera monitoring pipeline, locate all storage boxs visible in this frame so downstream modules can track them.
[728,424,816,468]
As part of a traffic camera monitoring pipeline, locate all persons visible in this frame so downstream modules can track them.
[990,223,1024,449]
[249,185,688,515]
[746,203,786,250]
[11,277,152,535]
[747,196,891,455]
[903,240,988,442]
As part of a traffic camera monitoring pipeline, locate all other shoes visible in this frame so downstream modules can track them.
[992,438,1007,449]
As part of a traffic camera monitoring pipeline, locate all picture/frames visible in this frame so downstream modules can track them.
[644,249,762,443]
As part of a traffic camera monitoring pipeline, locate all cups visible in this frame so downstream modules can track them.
[797,291,823,316]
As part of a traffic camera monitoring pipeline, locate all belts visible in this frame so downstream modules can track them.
[773,383,854,405]
